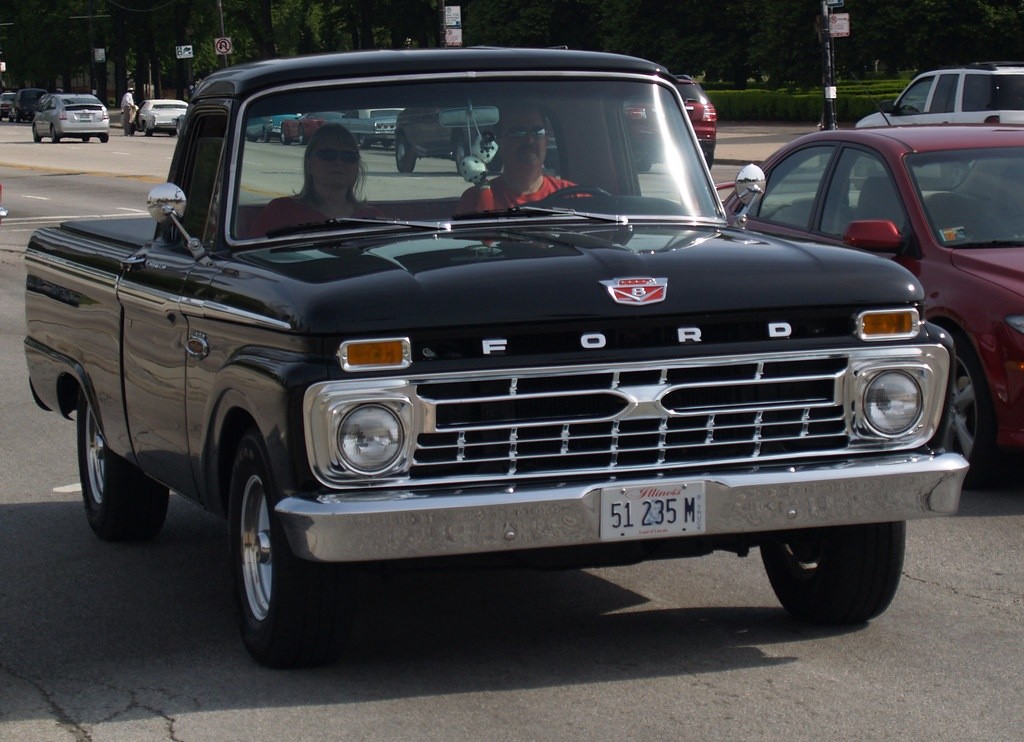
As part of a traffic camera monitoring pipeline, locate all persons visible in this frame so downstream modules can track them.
[245,122,382,238]
[122,87,135,137]
[459,100,594,219]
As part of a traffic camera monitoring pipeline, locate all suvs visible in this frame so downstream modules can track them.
[395,72,717,173]
[854,62,1024,130]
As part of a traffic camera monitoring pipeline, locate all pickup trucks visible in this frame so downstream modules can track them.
[23,45,972,670]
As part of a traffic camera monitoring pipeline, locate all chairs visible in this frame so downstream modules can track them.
[857,174,907,243]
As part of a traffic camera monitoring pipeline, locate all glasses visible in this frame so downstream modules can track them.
[315,148,362,161]
[506,126,550,138]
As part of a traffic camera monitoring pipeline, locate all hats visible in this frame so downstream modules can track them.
[127,87,134,90]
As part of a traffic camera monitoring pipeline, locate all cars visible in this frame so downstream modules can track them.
[121,99,190,136]
[8,87,47,122]
[725,122,1024,491]
[0,91,19,120]
[243,108,403,149]
[32,93,110,143]
